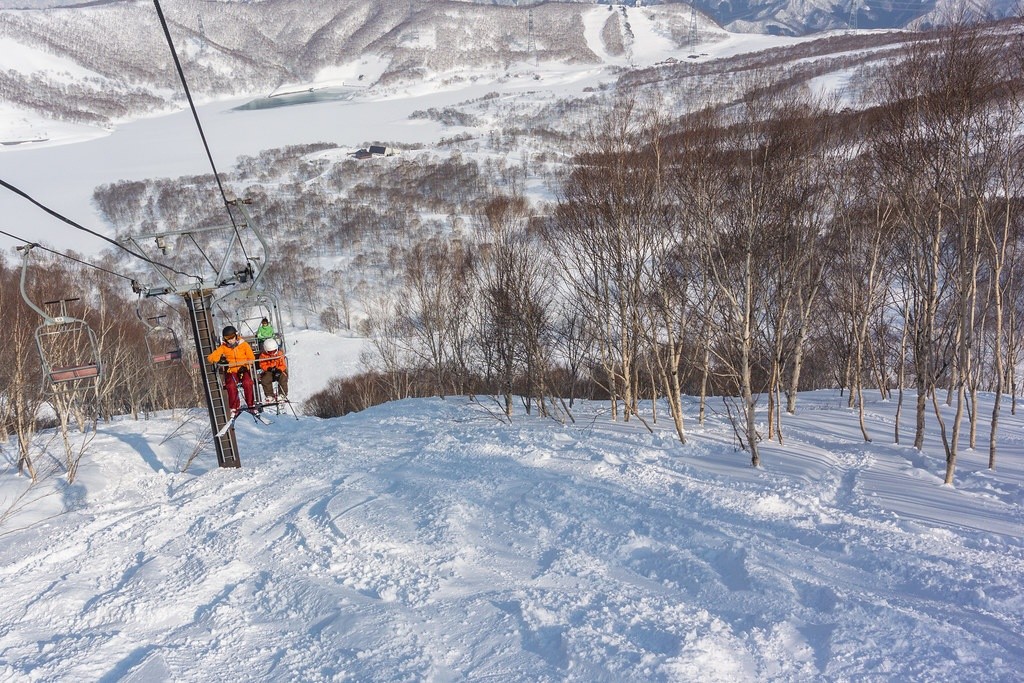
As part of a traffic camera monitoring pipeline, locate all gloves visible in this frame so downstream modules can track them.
[217,360,229,371]
[267,366,282,381]
[237,365,247,380]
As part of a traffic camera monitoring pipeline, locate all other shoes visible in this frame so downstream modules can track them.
[276,393,285,402]
[248,407,258,414]
[265,395,274,402]
[230,406,241,417]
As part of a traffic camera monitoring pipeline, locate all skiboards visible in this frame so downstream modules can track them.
[215,405,274,441]
[259,400,297,407]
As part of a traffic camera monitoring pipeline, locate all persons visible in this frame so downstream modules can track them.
[259,338,288,402]
[256,318,274,350]
[207,326,259,419]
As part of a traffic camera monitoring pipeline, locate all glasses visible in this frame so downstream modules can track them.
[262,322,267,324]
[224,334,236,340]
[268,350,277,354]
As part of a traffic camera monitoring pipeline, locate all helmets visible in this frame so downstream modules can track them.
[222,326,237,339]
[264,339,279,355]
